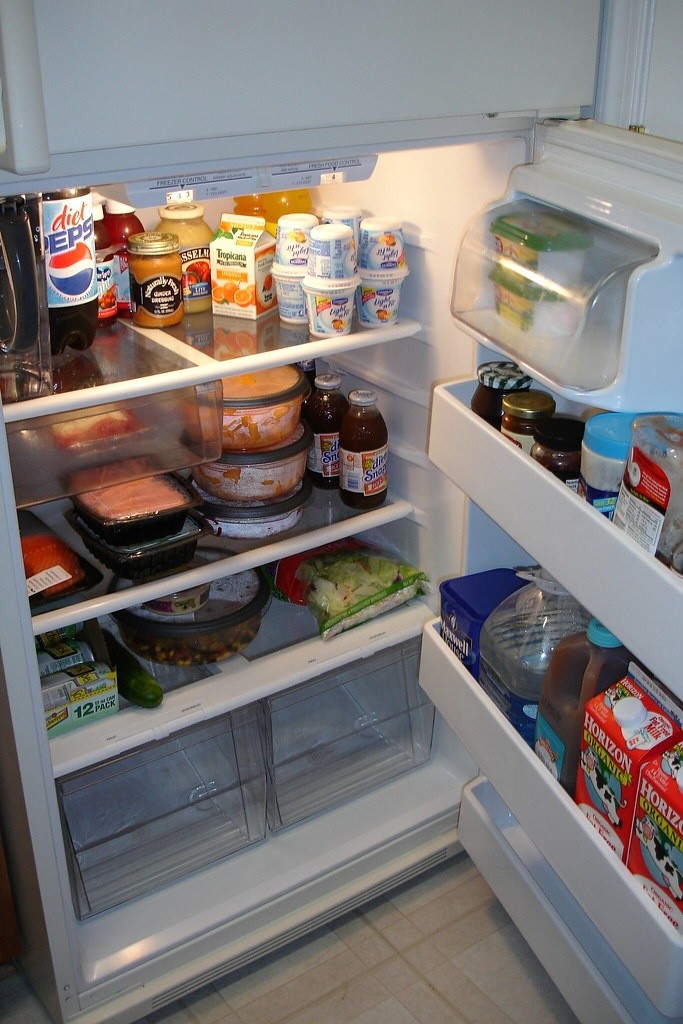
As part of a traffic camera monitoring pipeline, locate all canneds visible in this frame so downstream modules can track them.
[34,620,115,712]
[127,232,185,327]
[469,361,648,526]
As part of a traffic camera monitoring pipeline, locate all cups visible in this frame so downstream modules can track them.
[269,206,411,338]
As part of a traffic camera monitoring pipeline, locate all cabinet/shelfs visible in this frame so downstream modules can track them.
[2,316,436,784]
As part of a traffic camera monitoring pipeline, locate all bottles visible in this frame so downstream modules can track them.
[534,617,633,795]
[469,361,683,565]
[38,184,98,353]
[477,567,592,748]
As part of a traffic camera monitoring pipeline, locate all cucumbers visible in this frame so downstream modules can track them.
[99,629,164,709]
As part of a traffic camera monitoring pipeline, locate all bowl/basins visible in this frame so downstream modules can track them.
[105,547,271,667]
[64,363,314,580]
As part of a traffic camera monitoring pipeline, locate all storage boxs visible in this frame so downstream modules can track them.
[44,617,119,740]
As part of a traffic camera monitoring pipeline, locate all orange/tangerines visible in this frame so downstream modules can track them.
[211,280,256,307]
[213,328,257,353]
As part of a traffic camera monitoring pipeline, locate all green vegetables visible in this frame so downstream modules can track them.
[301,552,419,623]
